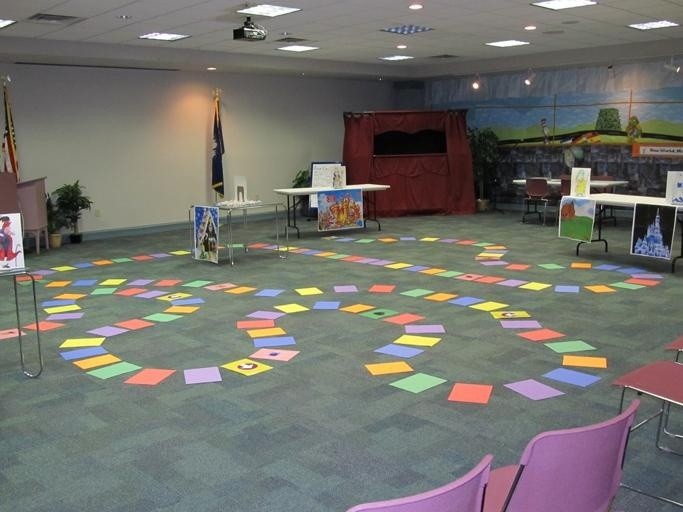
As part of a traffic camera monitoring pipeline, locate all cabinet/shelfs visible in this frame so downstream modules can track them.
[15,174,50,256]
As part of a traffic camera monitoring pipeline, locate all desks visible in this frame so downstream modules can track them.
[575,191,683,274]
[272,182,391,241]
[187,200,293,270]
[0,266,45,380]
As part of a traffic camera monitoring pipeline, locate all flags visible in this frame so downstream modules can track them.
[212,98,226,197]
[2,88,18,180]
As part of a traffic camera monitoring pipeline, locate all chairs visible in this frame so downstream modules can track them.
[509,173,631,229]
[344,452,495,512]
[483,397,642,511]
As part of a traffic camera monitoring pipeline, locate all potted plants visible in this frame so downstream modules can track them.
[44,192,70,249]
[49,179,96,245]
[472,126,505,212]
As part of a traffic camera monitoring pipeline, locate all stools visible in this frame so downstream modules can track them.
[662,332,683,439]
[609,356,683,509]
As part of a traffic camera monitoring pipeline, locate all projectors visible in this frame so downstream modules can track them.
[233,27,266,40]
